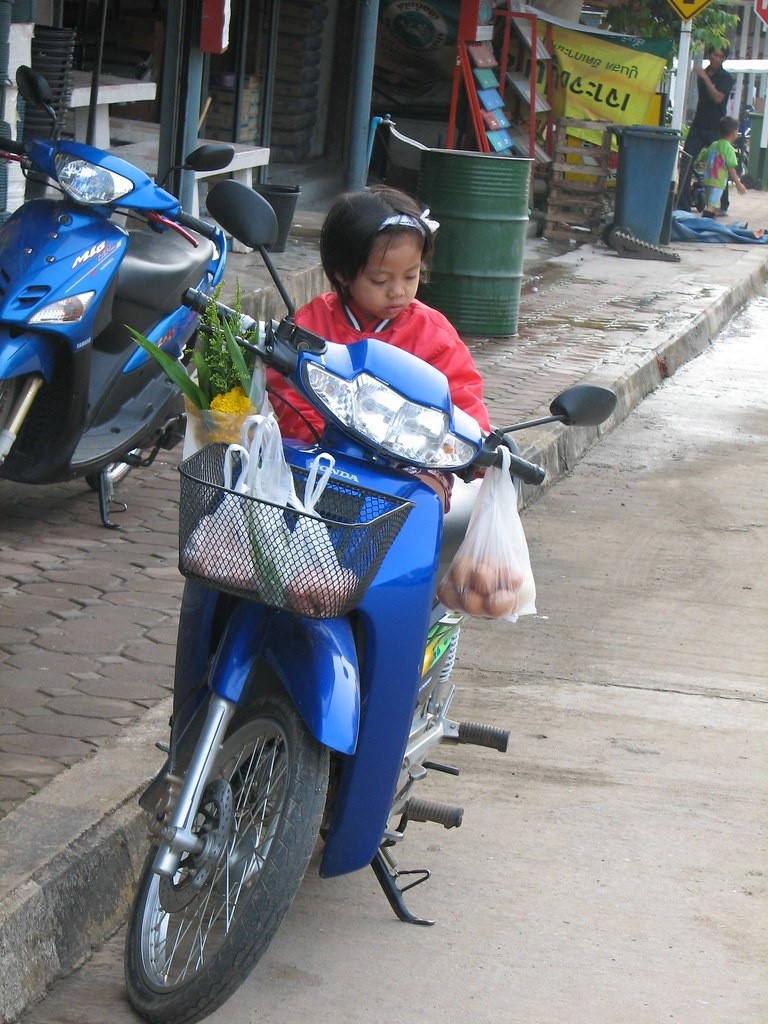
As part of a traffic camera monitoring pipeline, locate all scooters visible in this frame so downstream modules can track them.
[0,64,228,527]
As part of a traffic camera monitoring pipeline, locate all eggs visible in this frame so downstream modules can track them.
[435,552,523,617]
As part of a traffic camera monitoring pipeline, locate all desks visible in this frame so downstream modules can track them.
[67,71,156,150]
[103,139,270,253]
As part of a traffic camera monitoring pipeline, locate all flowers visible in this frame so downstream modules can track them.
[123,275,263,445]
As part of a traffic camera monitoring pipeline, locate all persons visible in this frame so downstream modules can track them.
[701,115,747,219]
[676,43,736,217]
[266,184,493,514]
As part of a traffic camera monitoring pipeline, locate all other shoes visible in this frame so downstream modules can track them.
[718,210,726,216]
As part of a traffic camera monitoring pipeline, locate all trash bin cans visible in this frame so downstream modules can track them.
[606,123,684,248]
[746,112,768,192]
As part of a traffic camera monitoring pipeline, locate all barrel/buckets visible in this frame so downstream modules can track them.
[421,147,536,337]
[253,183,302,253]
[25,24,77,202]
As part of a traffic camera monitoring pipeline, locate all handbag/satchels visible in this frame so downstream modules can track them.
[184,313,268,458]
[436,444,538,623]
[180,411,355,619]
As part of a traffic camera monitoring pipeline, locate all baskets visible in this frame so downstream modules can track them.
[176,441,416,619]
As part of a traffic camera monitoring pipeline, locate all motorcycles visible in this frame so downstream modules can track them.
[123,179,618,1024]
